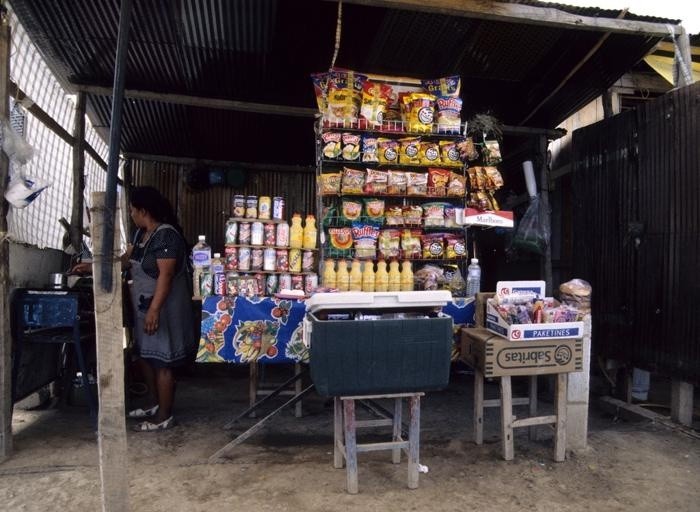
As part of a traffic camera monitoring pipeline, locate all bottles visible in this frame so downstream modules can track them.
[291,276,303,290]
[265,274,278,296]
[273,197,285,219]
[224,220,237,243]
[289,249,301,273]
[192,236,211,296]
[322,258,336,288]
[224,248,237,270]
[214,272,226,296]
[255,274,266,297]
[362,259,375,292]
[246,196,258,219]
[276,224,290,246]
[238,247,250,270]
[466,258,481,298]
[278,275,291,290]
[263,248,276,271]
[375,260,388,292]
[388,259,401,292]
[250,249,264,271]
[303,215,317,248]
[251,222,264,245]
[259,196,271,219]
[290,213,304,247]
[401,259,414,291]
[264,224,275,245]
[349,259,363,291]
[209,253,225,274]
[305,273,318,293]
[276,249,288,272]
[336,259,349,291]
[200,271,212,294]
[302,249,314,272]
[239,223,251,244]
[233,194,245,217]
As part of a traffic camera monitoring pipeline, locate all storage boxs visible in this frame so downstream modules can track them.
[486,297,584,341]
[460,328,584,378]
[301,289,452,395]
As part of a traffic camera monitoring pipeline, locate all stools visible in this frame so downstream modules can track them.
[248,361,302,419]
[333,392,425,494]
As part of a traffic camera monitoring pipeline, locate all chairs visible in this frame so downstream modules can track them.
[473,292,568,463]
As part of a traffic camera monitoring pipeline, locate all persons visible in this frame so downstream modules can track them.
[72,186,195,432]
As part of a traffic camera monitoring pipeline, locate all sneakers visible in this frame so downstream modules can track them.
[129,405,160,417]
[131,415,178,430]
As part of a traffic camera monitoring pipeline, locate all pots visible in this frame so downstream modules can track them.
[48,272,83,290]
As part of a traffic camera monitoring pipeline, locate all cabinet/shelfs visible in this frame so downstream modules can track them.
[315,71,469,259]
[191,216,475,462]
